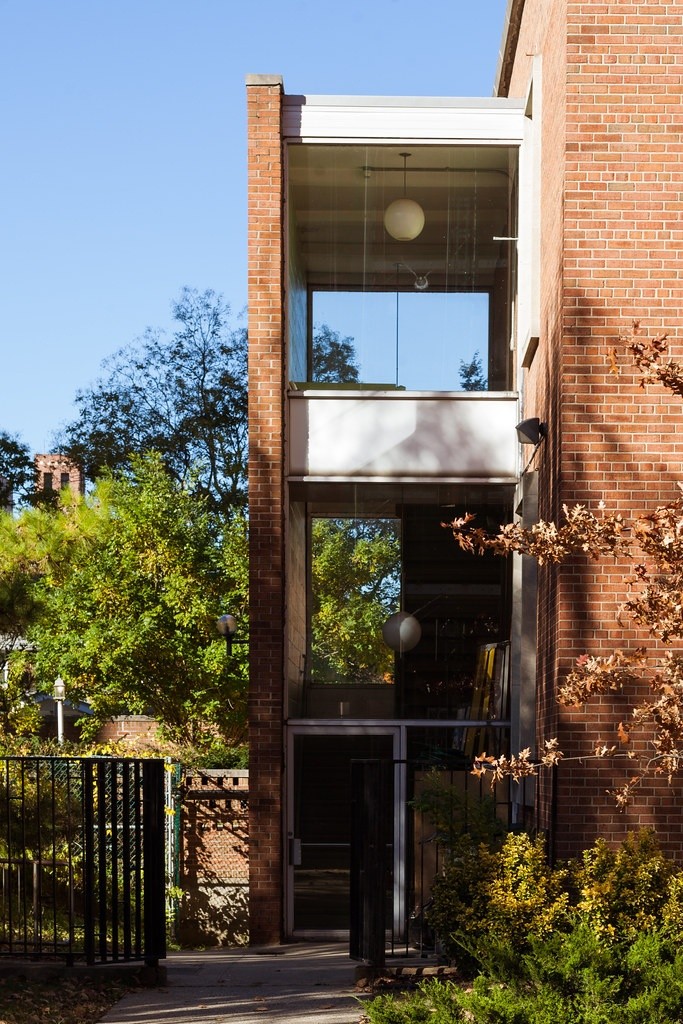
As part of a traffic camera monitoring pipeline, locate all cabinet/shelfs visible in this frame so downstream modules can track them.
[395,503,503,583]
[396,593,502,762]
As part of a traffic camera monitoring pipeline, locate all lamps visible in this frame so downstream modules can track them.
[381,612,421,652]
[517,416,548,445]
[381,152,426,241]
